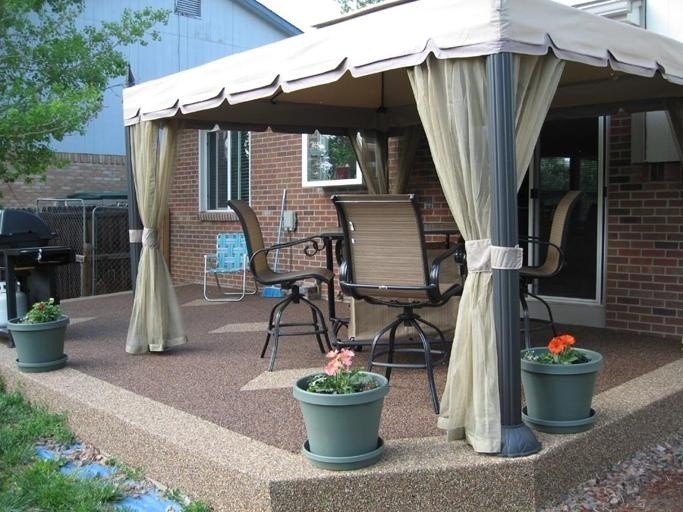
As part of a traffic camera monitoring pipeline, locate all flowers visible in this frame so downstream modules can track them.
[18,299,61,323]
[524,332,591,363]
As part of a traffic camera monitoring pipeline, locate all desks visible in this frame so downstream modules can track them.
[320,223,462,325]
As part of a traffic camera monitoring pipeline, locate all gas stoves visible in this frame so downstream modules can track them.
[0,209,75,271]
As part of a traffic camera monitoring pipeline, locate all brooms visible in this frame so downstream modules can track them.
[261,188,287,298]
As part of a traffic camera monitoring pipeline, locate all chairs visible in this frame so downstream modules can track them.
[517,188,587,339]
[203,233,258,302]
[225,198,337,371]
[330,194,467,416]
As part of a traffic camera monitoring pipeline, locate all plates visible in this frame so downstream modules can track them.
[518,407,599,436]
[15,356,69,372]
[300,436,390,471]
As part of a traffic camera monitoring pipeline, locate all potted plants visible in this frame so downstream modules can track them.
[290,371,390,471]
[327,139,354,180]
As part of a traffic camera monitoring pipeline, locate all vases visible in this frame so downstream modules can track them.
[521,346,600,435]
[7,315,71,373]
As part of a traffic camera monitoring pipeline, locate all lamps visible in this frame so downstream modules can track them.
[358,73,395,145]
